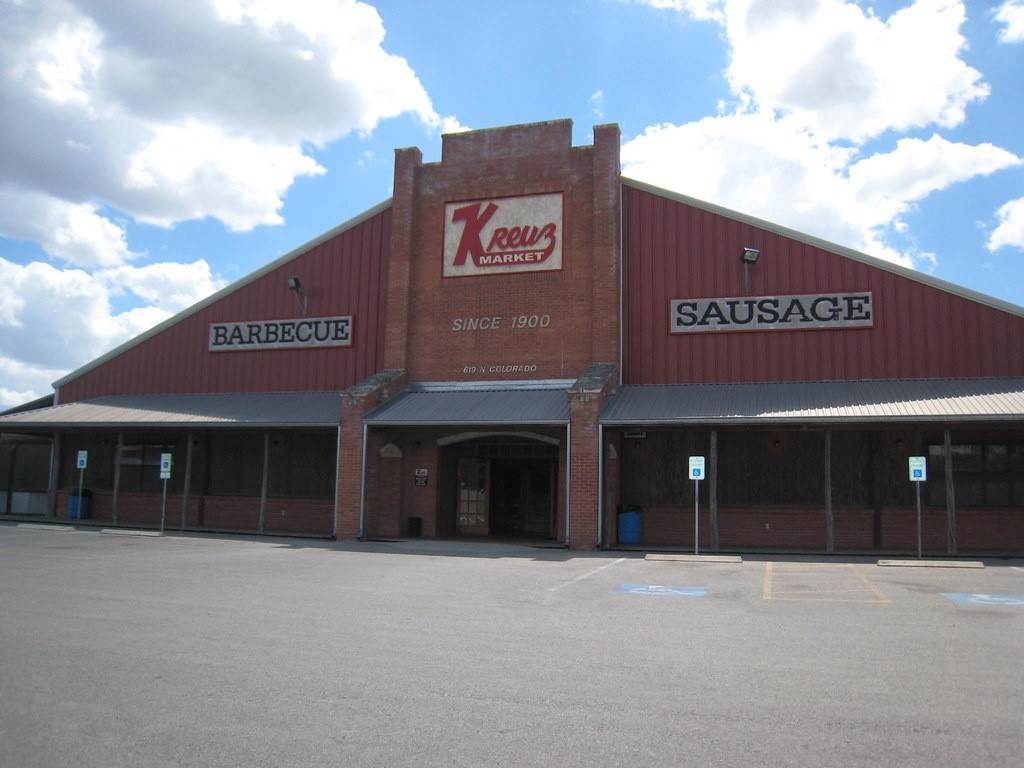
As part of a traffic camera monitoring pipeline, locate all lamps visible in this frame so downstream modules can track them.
[286,277,301,295]
[740,247,761,266]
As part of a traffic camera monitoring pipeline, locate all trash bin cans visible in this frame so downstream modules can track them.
[617,504,642,544]
[68,487,92,519]
